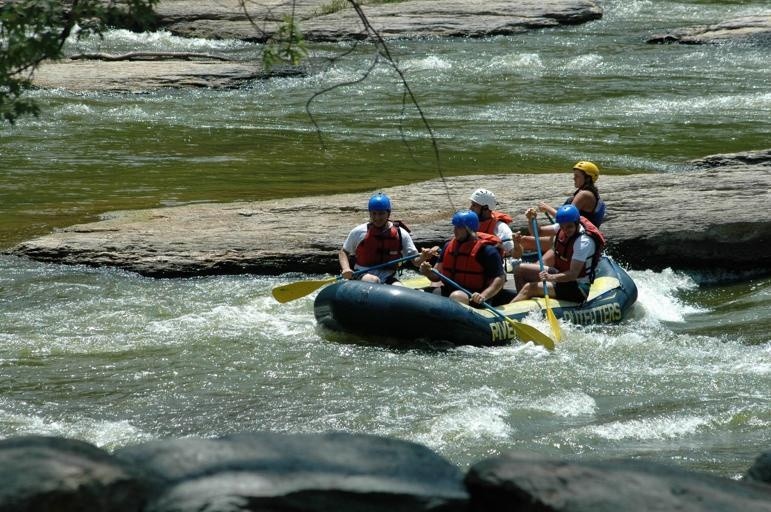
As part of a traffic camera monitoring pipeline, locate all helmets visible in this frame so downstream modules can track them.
[469,189,497,211]
[452,209,479,232]
[555,204,580,223]
[573,161,600,183]
[368,192,391,212]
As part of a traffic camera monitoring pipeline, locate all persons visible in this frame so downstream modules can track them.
[525,199,605,244]
[510,203,597,306]
[519,162,606,253]
[336,191,433,286]
[431,190,516,281]
[418,209,505,308]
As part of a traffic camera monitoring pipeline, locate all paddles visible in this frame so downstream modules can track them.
[427,264,555,353]
[529,209,561,343]
[271,248,422,305]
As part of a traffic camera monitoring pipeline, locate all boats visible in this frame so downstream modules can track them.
[315,246,641,346]
